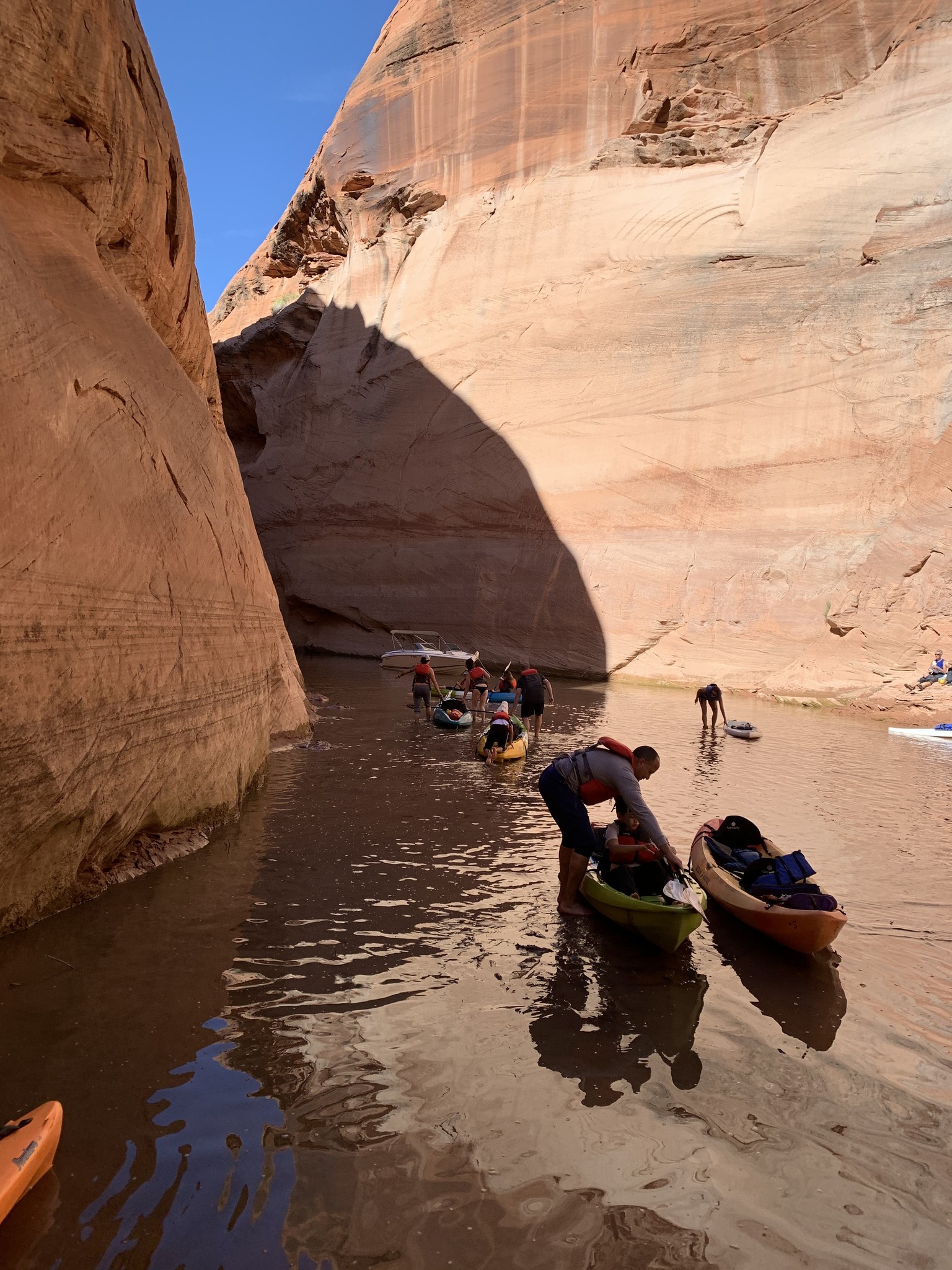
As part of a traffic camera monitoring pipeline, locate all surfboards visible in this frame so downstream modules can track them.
[723,720,763,738]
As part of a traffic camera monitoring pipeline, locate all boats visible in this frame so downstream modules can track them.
[579,821,708,954]
[0,1099,64,1225]
[431,686,523,703]
[478,713,528,762]
[888,726,952,739]
[379,629,481,676]
[724,720,763,739]
[434,698,474,726]
[690,815,853,956]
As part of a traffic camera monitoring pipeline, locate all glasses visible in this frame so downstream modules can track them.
[711,692,717,696]
[504,675,506,677]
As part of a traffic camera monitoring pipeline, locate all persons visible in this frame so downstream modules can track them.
[695,684,729,730]
[509,664,554,739]
[484,700,514,767]
[904,649,949,691]
[941,660,952,686]
[438,658,513,723]
[538,745,685,916]
[395,654,443,725]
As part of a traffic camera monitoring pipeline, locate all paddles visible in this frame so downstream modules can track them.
[486,660,511,703]
[444,651,479,700]
[663,864,710,923]
[406,704,518,716]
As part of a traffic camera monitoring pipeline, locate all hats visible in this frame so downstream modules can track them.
[421,654,431,660]
[463,669,469,675]
